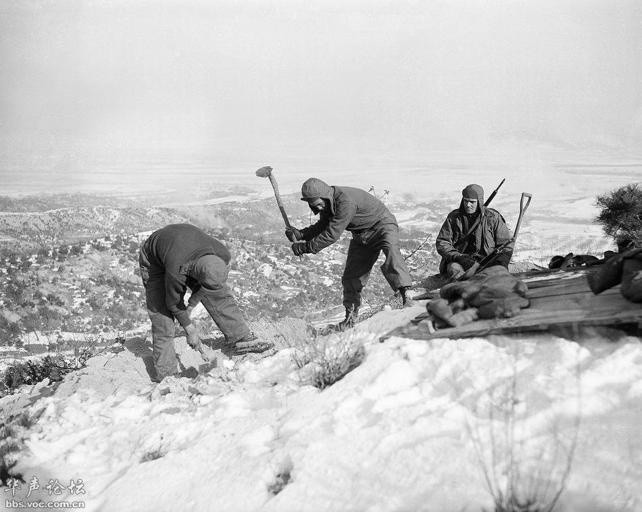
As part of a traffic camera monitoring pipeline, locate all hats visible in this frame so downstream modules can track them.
[459,184,486,213]
[192,255,228,294]
[301,178,334,216]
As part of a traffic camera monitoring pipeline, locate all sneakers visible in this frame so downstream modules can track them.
[234,332,274,354]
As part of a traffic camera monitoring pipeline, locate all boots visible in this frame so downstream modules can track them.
[326,306,358,330]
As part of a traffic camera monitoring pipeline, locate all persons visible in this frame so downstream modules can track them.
[285,178,412,335]
[435,184,515,283]
[138,223,275,382]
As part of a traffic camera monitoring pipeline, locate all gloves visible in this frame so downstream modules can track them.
[292,241,313,256]
[285,226,304,242]
[454,254,475,271]
